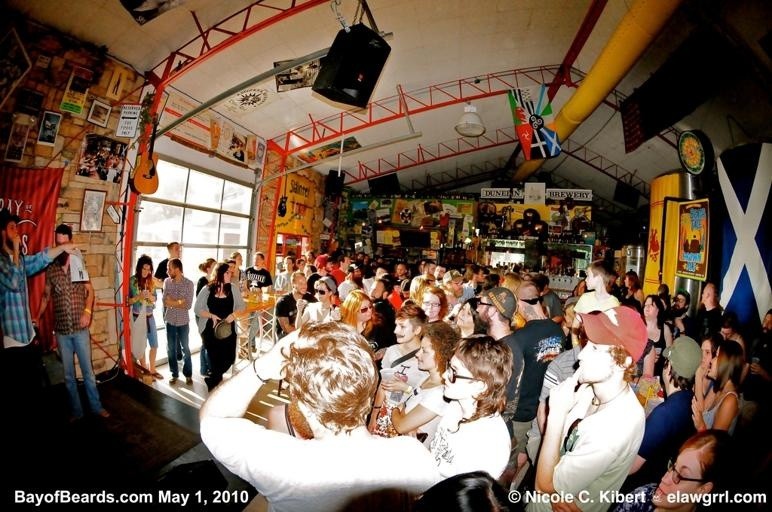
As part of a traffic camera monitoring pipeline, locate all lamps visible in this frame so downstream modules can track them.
[454,100,487,139]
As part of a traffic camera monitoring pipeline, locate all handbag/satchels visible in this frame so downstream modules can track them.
[213,318,233,340]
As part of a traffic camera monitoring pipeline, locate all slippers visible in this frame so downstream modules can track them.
[147,368,164,380]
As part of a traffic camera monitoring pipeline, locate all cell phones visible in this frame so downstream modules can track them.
[751,357,760,374]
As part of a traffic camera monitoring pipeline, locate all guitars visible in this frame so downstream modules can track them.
[131,122,160,194]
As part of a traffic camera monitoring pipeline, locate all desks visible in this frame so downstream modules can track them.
[232,289,291,376]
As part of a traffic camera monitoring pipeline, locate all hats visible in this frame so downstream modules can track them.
[662,335,702,380]
[576,305,649,362]
[487,287,516,319]
[675,287,691,302]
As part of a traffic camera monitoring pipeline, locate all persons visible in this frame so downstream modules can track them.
[77,149,122,182]
[32,224,110,424]
[131,237,772,512]
[1,211,88,398]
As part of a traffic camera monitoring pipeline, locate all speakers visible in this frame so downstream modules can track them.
[325,170,345,197]
[311,24,391,109]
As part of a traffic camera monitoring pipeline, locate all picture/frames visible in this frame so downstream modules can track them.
[79,187,107,233]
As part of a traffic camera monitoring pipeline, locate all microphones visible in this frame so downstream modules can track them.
[15,234,25,258]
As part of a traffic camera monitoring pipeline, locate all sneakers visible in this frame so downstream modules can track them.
[168,376,178,384]
[185,376,193,385]
[95,408,111,418]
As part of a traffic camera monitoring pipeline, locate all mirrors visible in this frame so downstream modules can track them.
[480,237,594,280]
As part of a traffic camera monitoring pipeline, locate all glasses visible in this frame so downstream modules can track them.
[314,288,328,295]
[359,305,374,314]
[667,457,702,485]
[445,358,483,385]
[421,301,442,307]
[478,301,492,307]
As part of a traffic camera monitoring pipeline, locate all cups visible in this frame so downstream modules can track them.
[244,287,272,303]
[379,368,397,382]
[750,357,759,364]
